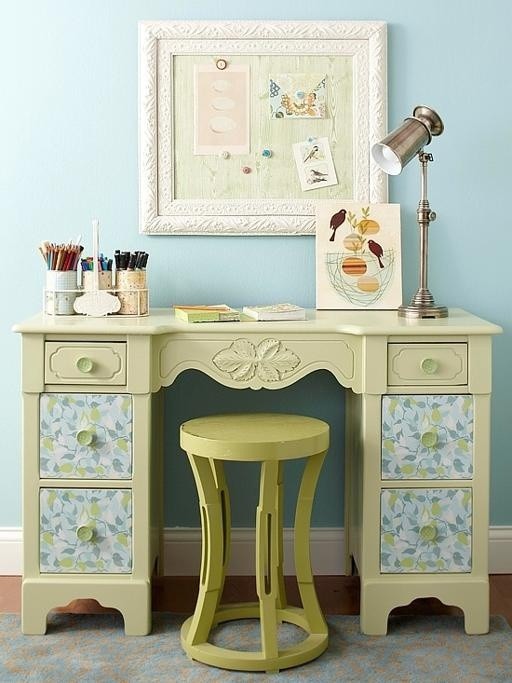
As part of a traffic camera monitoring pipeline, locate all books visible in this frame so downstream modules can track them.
[171,303,241,323]
[243,301,307,320]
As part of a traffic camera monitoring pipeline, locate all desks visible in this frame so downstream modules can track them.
[11,309,501,635]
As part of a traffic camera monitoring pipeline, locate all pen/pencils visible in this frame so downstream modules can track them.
[173,305,230,311]
[39,234,149,272]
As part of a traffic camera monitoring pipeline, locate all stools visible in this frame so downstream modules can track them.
[177,412,330,674]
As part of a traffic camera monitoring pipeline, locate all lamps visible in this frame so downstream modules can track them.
[370,105,452,319]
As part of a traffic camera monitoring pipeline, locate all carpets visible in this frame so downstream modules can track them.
[0,612,512,683]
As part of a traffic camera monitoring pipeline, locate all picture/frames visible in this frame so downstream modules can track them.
[137,18,389,238]
